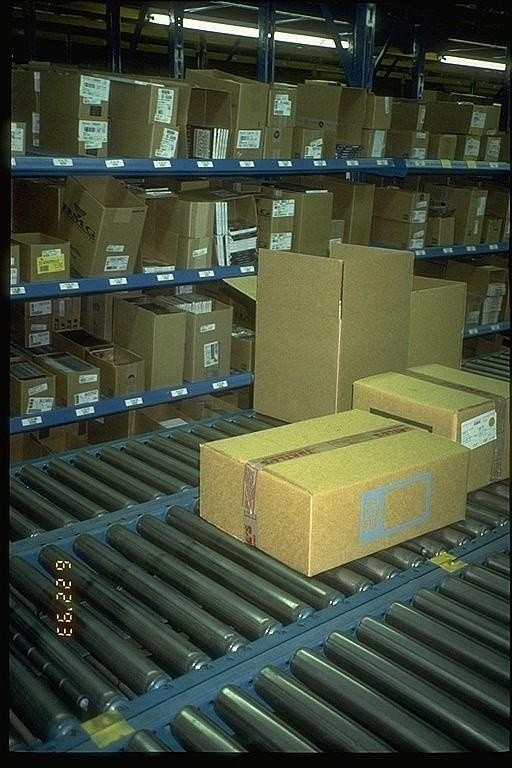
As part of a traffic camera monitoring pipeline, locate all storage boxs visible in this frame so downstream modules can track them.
[197,405,471,576]
[349,362,512,496]
[14,61,511,477]
